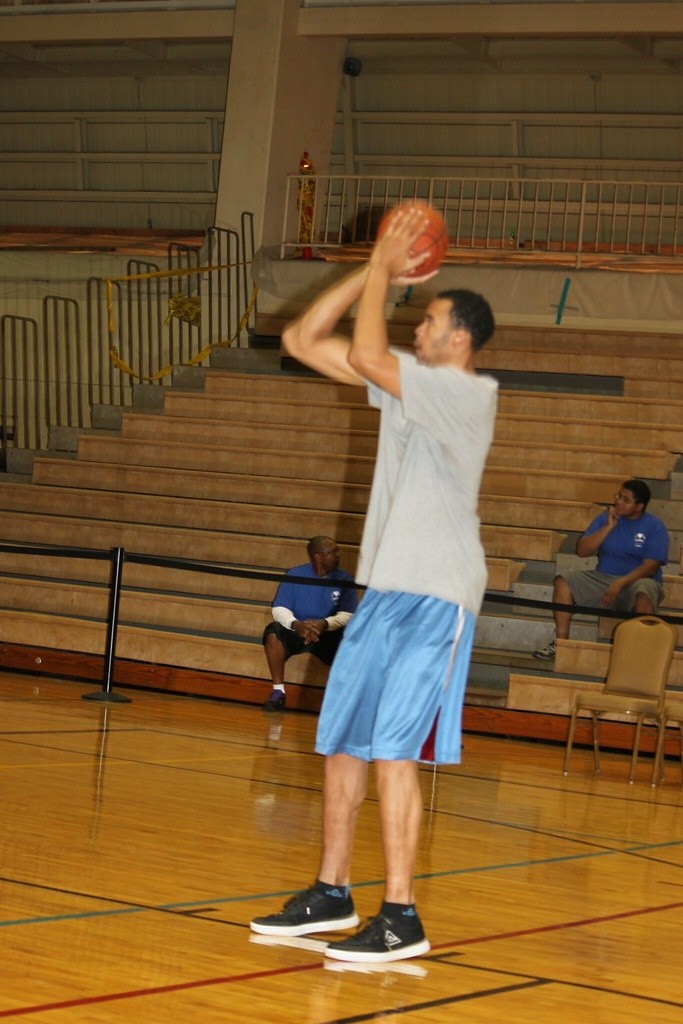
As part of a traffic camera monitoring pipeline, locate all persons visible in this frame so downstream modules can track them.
[248,209,501,961]
[531,479,670,661]
[262,535,359,712]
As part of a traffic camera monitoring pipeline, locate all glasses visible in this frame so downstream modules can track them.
[315,548,340,556]
[614,493,635,504]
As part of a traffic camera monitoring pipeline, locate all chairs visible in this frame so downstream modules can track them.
[562,614,677,789]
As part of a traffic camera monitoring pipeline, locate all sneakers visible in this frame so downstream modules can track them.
[533,639,557,660]
[249,887,360,935]
[263,689,287,711]
[323,913,430,963]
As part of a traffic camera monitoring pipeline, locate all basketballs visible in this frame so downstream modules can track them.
[376,199,448,278]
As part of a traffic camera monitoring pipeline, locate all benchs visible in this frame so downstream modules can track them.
[1,313,681,760]
